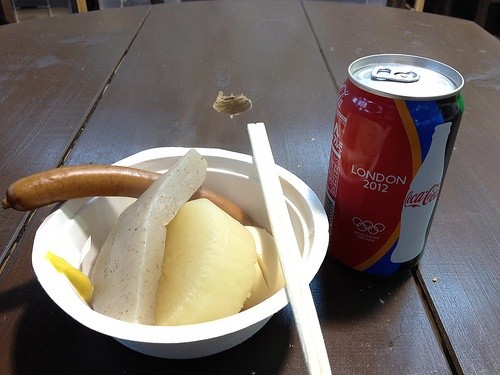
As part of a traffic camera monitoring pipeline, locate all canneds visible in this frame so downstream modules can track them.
[323,54,465,275]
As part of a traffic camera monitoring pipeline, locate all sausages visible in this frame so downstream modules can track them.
[0,166,266,228]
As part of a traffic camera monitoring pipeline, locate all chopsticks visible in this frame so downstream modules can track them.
[247,122,333,375]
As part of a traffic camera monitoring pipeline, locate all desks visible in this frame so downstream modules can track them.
[0,0,500,375]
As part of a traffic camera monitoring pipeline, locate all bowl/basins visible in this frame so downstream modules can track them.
[32,146,329,360]
